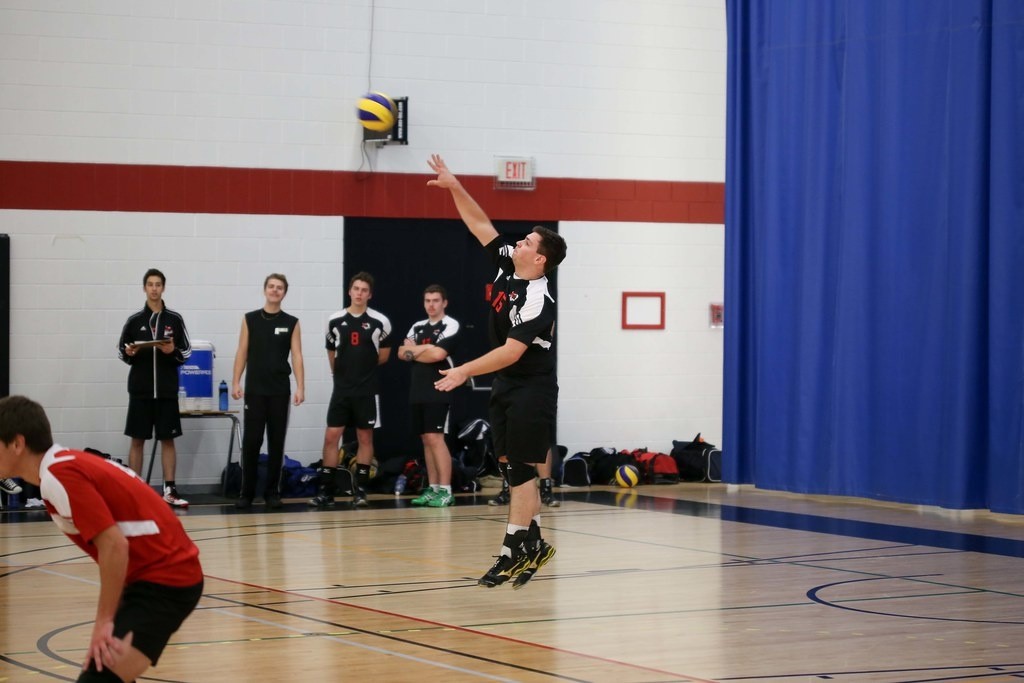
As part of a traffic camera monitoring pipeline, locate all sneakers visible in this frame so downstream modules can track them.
[540,487,561,508]
[508,539,558,590]
[476,548,528,589]
[163,485,190,508]
[410,487,440,506]
[309,492,337,507]
[428,489,457,507]
[487,490,510,507]
[350,492,367,508]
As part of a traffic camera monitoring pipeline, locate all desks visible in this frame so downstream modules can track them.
[145,410,244,501]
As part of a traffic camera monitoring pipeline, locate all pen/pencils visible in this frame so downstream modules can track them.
[125,343,135,354]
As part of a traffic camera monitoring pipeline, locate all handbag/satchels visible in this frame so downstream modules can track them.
[623,448,681,487]
[255,441,386,496]
[559,445,643,487]
[673,432,722,485]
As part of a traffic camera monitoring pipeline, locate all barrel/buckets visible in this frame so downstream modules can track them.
[177,339,216,411]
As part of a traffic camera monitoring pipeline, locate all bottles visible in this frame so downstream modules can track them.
[178,387,186,411]
[395,475,406,495]
[219,380,228,411]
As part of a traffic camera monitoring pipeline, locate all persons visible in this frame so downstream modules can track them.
[488,447,560,508]
[231,272,305,508]
[427,154,568,589]
[117,269,192,506]
[0,394,204,683]
[397,285,462,506]
[307,271,392,504]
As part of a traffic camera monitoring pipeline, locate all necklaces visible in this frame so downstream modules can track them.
[260,309,282,319]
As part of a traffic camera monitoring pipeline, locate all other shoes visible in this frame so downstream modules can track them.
[235,494,252,510]
[263,495,283,509]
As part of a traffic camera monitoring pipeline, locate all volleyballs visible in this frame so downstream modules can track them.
[358,91,399,132]
